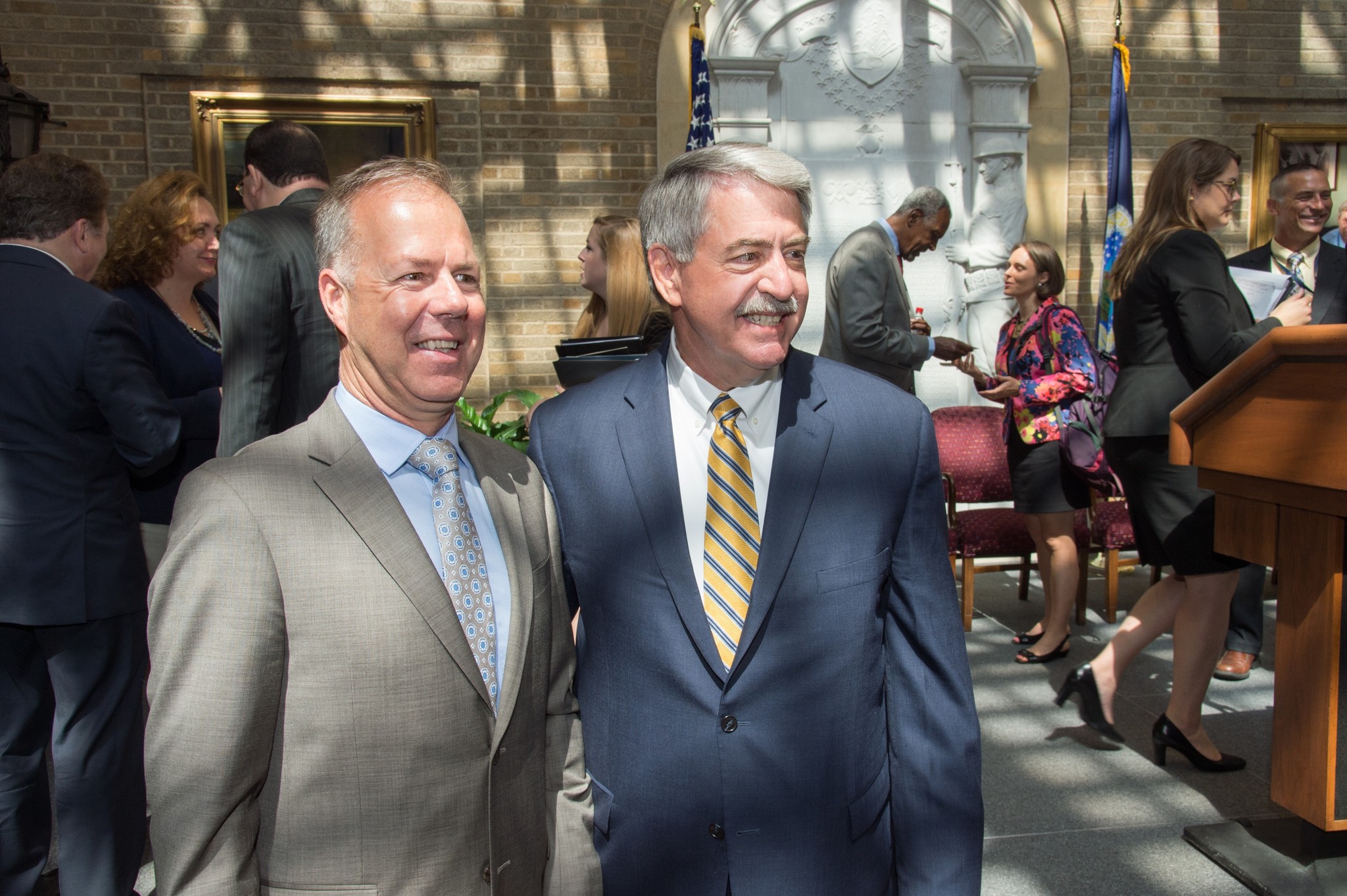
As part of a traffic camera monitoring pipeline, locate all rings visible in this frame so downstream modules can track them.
[964,364,969,366]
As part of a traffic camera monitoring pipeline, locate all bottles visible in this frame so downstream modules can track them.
[910,307,924,335]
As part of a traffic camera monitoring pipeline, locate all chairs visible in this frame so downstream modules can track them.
[1077,462,1160,625]
[931,406,1039,631]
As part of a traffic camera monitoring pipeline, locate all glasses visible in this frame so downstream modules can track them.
[1195,176,1239,196]
[235,173,251,196]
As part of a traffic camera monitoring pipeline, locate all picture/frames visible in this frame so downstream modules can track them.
[1246,123,1347,250]
[190,91,435,245]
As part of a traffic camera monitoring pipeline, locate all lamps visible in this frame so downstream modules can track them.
[0,57,50,171]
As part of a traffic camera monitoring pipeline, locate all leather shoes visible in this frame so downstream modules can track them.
[1214,647,1260,678]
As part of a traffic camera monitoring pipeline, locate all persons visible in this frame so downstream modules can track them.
[0,120,339,896]
[1212,161,1347,680]
[143,157,603,896]
[1054,136,1312,772]
[945,137,1028,408]
[818,187,975,395]
[524,214,672,431]
[528,143,984,896]
[951,241,1097,664]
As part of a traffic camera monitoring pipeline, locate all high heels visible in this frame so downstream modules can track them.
[1152,712,1246,772]
[1016,633,1071,664]
[1053,662,1124,743]
[1011,631,1047,644]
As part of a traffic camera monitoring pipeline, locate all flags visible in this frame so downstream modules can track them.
[1096,34,1134,355]
[686,25,717,152]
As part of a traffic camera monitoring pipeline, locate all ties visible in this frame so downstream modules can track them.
[406,437,499,721]
[1282,252,1306,304]
[897,254,904,276]
[700,391,761,674]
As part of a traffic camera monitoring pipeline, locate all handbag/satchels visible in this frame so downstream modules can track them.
[1037,304,1124,496]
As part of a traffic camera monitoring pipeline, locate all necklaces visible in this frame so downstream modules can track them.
[150,280,222,355]
